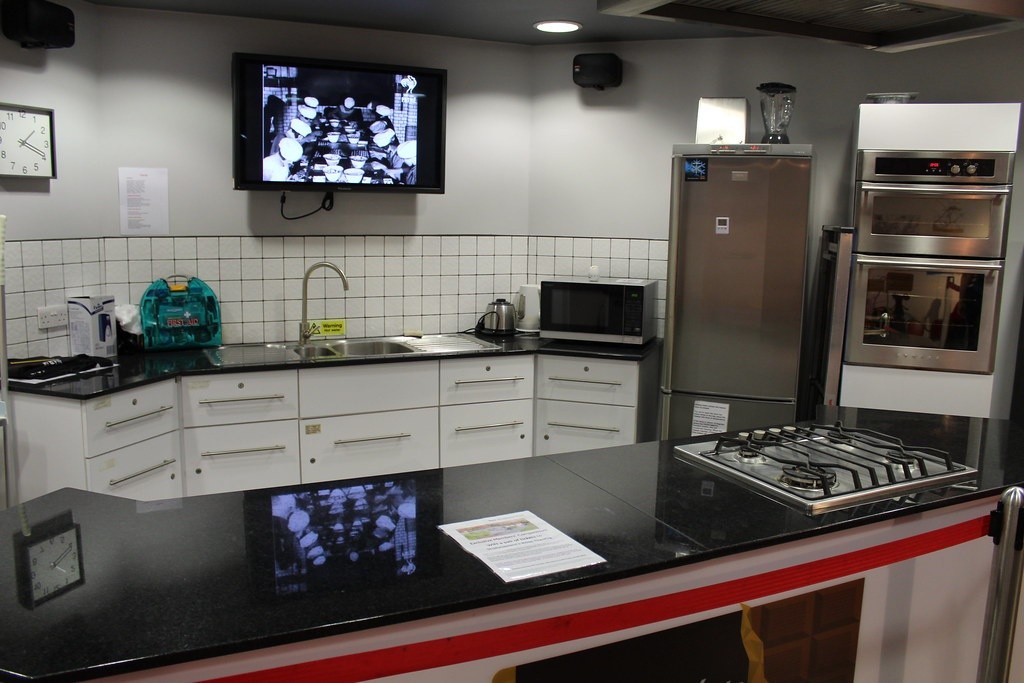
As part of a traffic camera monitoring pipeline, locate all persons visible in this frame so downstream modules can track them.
[374,105,399,146]
[385,140,417,184]
[353,483,403,562]
[304,96,319,108]
[287,106,322,144]
[370,128,403,179]
[270,119,311,155]
[263,137,303,181]
[332,97,356,120]
[272,494,326,565]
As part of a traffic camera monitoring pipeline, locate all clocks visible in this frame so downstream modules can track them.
[19,520,85,610]
[0,103,57,181]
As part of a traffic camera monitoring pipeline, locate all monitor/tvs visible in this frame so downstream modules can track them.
[230,52,448,193]
[244,466,443,604]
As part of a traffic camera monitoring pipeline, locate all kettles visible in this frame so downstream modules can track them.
[516,285,540,332]
[484,298,516,331]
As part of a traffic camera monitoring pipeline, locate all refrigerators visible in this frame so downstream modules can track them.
[656,143,817,440]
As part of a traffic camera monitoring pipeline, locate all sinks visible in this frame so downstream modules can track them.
[328,339,416,357]
[289,345,341,360]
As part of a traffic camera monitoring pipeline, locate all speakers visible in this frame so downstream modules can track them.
[573,54,622,88]
[0,0,75,48]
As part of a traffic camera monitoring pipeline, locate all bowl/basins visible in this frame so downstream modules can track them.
[327,132,341,143]
[327,486,369,514]
[344,125,359,143]
[323,154,368,183]
[329,119,341,127]
[332,520,363,544]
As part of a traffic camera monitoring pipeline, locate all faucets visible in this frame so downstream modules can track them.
[299,262,350,345]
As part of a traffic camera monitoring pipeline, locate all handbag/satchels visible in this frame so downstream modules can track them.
[139,274,223,350]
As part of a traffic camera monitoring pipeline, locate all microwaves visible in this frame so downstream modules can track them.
[540,278,659,344]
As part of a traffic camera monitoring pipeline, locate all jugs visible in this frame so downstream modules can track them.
[755,82,797,143]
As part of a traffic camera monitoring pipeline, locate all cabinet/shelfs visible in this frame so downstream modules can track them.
[9,337,665,504]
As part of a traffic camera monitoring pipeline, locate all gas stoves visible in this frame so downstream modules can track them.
[673,420,978,516]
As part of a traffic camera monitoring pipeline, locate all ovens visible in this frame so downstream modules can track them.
[845,148,1017,374]
